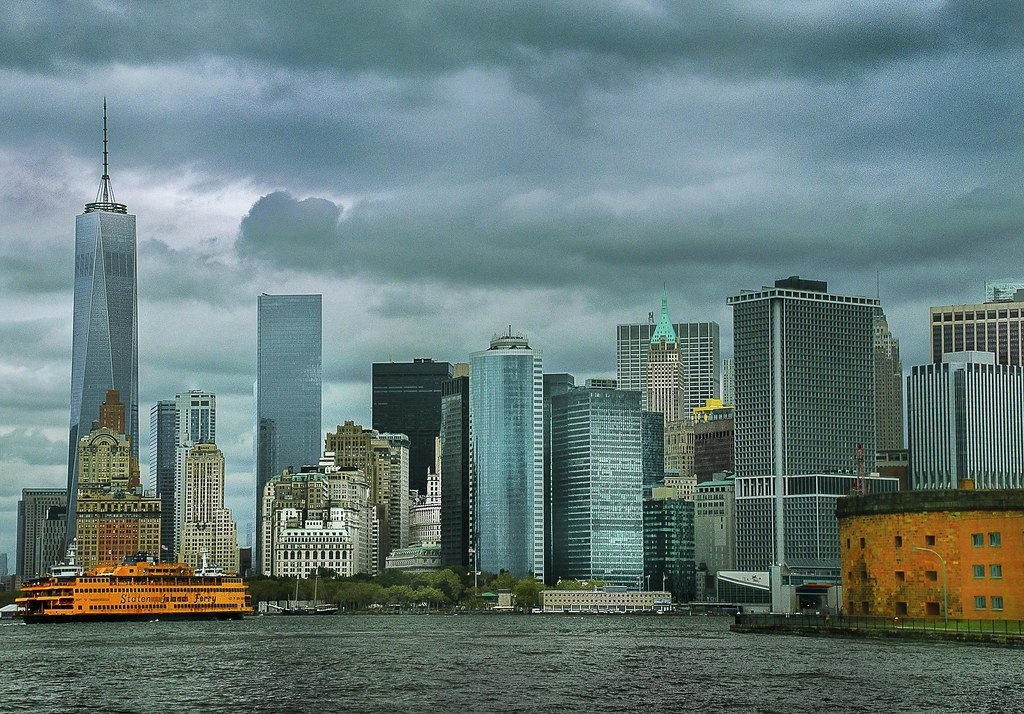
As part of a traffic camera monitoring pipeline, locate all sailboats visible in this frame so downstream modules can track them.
[265,566,339,615]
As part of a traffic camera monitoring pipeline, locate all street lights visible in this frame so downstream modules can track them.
[912,546,948,624]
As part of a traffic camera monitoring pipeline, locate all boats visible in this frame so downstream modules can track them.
[13,536,254,621]
